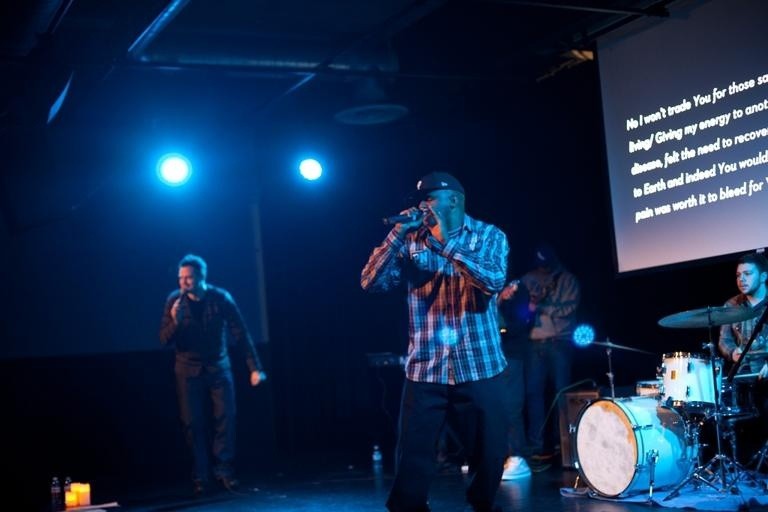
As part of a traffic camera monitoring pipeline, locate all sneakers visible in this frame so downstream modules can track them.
[501,456,533,480]
[530,455,553,472]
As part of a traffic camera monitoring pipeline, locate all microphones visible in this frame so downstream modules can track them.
[383,210,423,225]
[179,288,189,304]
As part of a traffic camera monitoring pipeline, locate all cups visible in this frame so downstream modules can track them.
[64,482,91,507]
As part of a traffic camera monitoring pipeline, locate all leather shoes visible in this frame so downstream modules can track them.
[193,477,249,496]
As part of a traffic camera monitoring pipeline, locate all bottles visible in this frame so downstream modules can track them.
[49,476,62,509]
[500,280,521,300]
[371,446,384,476]
[64,478,71,491]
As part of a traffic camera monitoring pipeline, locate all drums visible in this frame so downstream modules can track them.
[661,354,723,410]
[705,413,762,470]
[570,397,699,499]
[636,381,664,399]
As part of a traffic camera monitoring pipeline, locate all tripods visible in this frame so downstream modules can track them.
[706,383,765,494]
[663,326,767,501]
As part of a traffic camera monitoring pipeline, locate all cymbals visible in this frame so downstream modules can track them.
[736,349,767,356]
[658,306,761,329]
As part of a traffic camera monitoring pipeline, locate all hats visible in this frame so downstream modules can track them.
[408,171,465,195]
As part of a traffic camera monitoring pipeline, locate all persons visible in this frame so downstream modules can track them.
[716,254,768,383]
[360,170,515,512]
[159,254,269,496]
[493,245,583,461]
